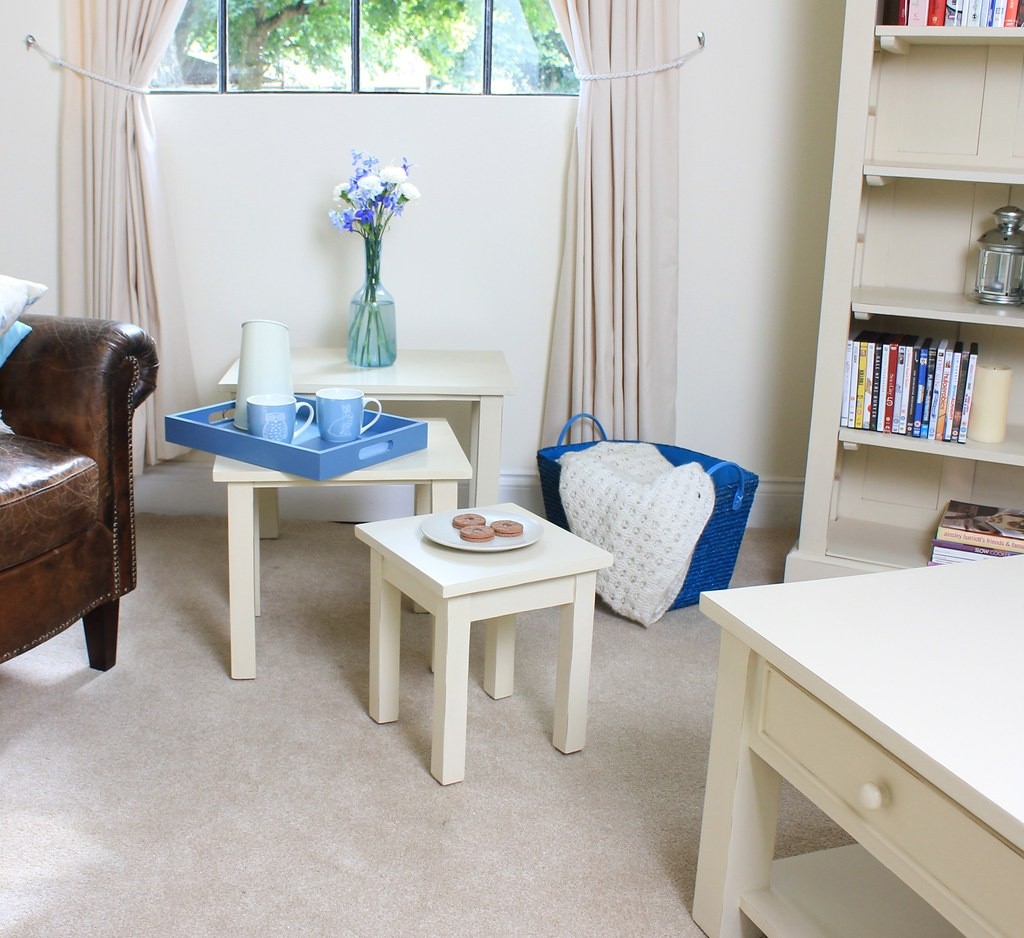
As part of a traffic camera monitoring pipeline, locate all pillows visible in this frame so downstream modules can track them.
[0,273,49,365]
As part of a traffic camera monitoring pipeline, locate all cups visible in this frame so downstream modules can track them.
[233,319,295,431]
[315,387,382,442]
[246,394,314,445]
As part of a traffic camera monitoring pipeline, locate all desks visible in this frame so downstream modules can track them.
[353,502,615,786]
[212,417,475,681]
[691,556,1024,938]
[216,345,516,539]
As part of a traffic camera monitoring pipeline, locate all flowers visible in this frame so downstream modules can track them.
[327,147,421,256]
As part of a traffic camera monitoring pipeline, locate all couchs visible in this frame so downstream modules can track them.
[0,314,161,672]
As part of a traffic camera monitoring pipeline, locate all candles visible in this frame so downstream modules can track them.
[968,361,1012,444]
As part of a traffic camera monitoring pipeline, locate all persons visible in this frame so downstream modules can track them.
[953,410,962,428]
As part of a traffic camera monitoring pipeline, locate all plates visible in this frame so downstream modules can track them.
[420,508,544,551]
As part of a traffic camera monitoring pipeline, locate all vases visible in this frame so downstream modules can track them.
[348,263,398,367]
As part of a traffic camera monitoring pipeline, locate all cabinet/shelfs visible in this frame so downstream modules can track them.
[783,0,1024,575]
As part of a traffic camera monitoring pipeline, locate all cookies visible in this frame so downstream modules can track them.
[452,513,523,542]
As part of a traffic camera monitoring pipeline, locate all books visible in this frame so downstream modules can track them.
[882,0,1024,27]
[840,330,979,445]
[927,500,1024,566]
